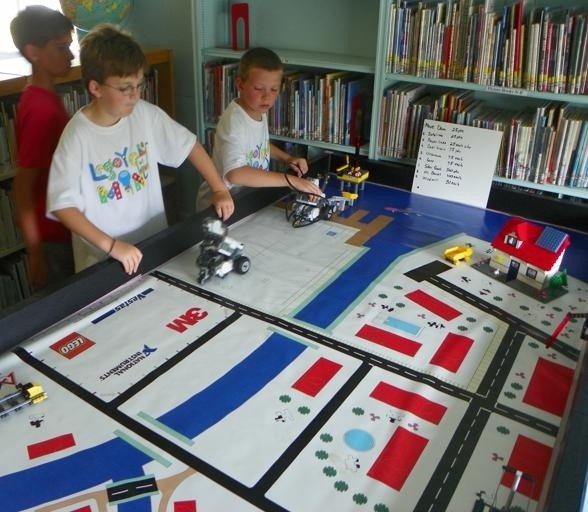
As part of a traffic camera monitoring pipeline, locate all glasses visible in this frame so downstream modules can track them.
[99,80,147,96]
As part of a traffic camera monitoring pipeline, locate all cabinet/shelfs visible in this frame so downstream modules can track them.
[1,48,174,302]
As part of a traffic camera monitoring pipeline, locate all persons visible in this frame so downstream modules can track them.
[40,20,239,277]
[6,3,79,295]
[192,46,326,214]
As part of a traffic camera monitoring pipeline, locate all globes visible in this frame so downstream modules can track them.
[59,0,134,33]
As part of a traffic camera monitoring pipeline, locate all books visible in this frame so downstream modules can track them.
[0,78,92,309]
[200,62,588,204]
[139,65,159,106]
[385,1,588,95]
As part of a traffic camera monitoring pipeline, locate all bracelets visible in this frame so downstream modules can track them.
[104,238,116,259]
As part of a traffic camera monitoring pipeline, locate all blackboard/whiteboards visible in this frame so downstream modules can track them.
[412,118,505,208]
